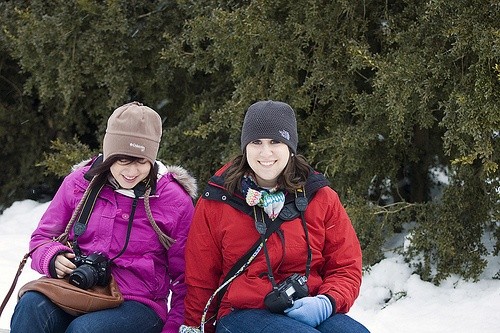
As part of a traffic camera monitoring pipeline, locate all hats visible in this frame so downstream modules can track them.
[241,100,298,155]
[102,102,162,169]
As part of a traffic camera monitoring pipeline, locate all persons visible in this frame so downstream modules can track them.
[9,102,198,333]
[184,100,370,333]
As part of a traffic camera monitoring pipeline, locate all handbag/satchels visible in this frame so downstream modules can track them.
[16,272,124,316]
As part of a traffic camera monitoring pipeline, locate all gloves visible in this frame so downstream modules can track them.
[283,294,332,327]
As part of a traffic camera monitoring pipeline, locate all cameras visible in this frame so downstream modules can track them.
[69,251,111,290]
[264,272,310,313]
